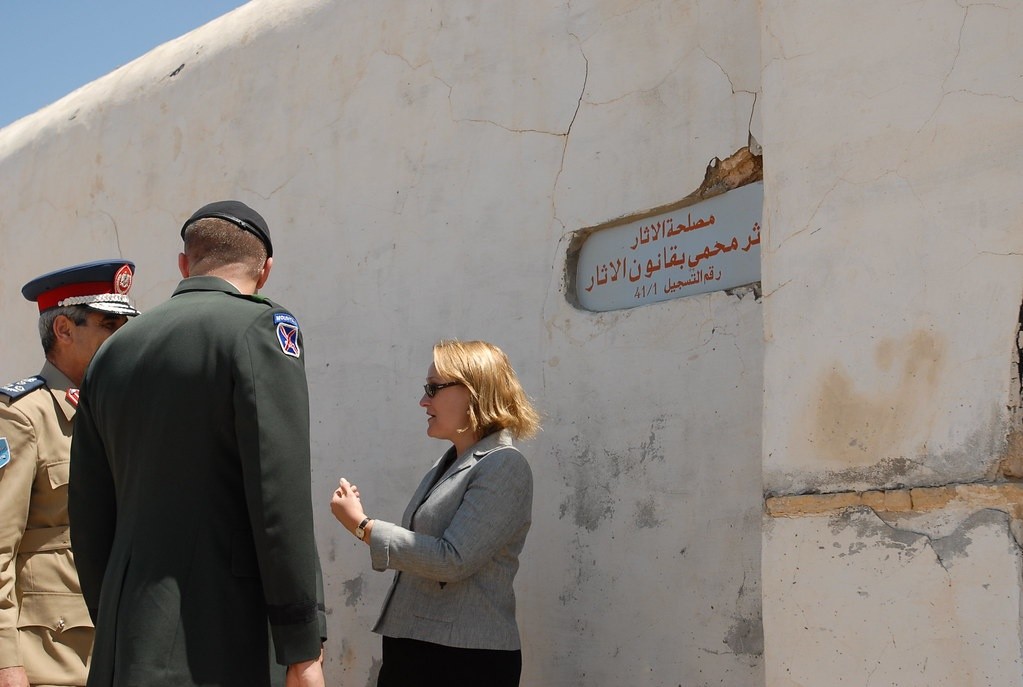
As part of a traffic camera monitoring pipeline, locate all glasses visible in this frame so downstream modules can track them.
[424,381,467,399]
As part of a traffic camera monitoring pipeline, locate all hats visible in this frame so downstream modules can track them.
[21,260,143,316]
[181,201,273,259]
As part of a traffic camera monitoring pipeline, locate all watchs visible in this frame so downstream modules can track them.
[355,518,372,540]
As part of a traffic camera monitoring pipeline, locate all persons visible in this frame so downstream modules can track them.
[330,339,539,687]
[67,201,326,687]
[0,258,141,687]
